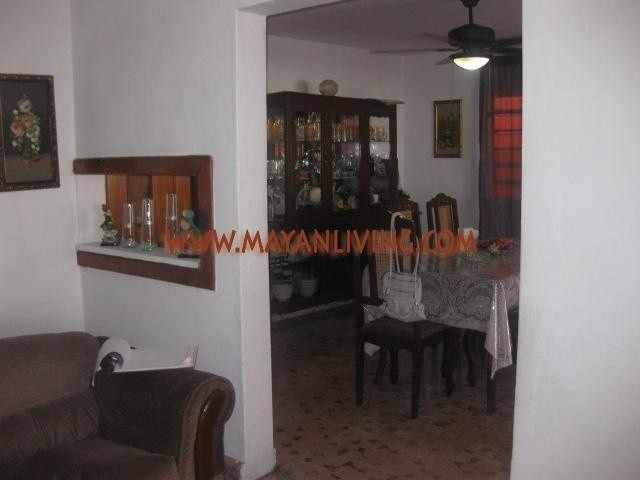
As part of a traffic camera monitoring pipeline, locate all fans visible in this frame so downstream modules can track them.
[366,0,521,67]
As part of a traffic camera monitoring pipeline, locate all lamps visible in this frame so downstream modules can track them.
[449,50,493,74]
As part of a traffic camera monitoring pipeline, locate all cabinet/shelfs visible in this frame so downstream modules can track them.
[266,90,404,326]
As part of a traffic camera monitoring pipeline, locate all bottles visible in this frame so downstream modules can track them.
[121,202,136,248]
[165,194,183,258]
[267,184,284,222]
[141,199,159,252]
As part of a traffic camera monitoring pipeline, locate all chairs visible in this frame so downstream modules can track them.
[350,205,457,419]
[372,197,478,398]
[425,192,489,388]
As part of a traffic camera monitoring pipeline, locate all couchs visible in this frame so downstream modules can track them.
[0,327,240,479]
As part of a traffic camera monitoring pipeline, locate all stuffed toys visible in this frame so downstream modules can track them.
[99,203,120,248]
[169,209,203,260]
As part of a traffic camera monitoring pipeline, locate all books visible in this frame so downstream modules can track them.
[91,337,199,388]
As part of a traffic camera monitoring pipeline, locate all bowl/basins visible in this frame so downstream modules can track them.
[272,276,319,303]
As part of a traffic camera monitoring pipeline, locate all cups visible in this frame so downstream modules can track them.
[268,111,388,182]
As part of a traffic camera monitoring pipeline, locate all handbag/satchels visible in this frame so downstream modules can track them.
[377,270,428,324]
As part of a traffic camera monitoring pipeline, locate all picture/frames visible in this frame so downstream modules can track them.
[0,71,61,194]
[431,97,464,160]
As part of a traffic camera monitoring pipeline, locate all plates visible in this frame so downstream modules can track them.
[477,240,519,256]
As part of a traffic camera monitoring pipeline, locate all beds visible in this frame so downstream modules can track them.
[373,237,520,412]
